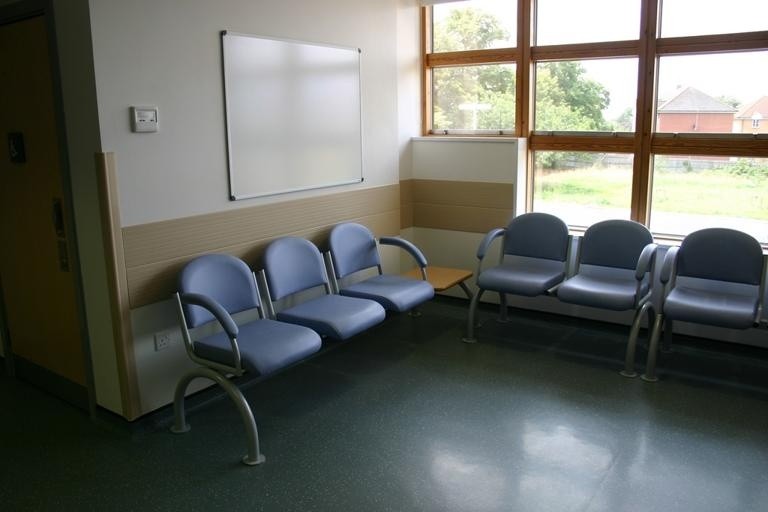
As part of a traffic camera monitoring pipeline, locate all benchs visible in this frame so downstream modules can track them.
[464,211,657,376]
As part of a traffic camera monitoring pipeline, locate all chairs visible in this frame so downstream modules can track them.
[642,228,767,384]
[166,220,431,467]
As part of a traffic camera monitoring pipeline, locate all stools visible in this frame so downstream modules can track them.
[395,265,483,331]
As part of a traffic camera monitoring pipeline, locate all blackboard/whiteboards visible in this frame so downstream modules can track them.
[221,30,363,201]
